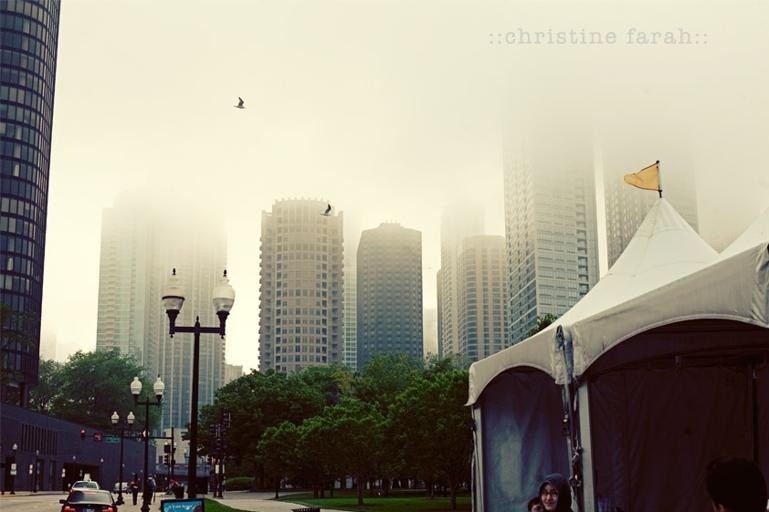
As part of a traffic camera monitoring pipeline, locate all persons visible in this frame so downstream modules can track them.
[527,496,544,512]
[130,472,141,505]
[172,480,184,498]
[539,473,574,512]
[165,477,178,494]
[147,472,157,506]
[704,456,768,511]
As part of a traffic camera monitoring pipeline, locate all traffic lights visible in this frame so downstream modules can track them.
[80,429,85,440]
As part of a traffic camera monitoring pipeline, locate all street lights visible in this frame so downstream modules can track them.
[110,264,237,511]
[10,443,105,495]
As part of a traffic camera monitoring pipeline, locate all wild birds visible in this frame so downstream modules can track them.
[233,97,246,109]
[320,204,332,216]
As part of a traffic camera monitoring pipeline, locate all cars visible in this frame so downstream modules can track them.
[58,488,124,512]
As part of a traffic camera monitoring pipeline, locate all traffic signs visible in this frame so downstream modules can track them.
[105,435,120,444]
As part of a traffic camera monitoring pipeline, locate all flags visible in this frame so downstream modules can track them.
[620,162,662,193]
